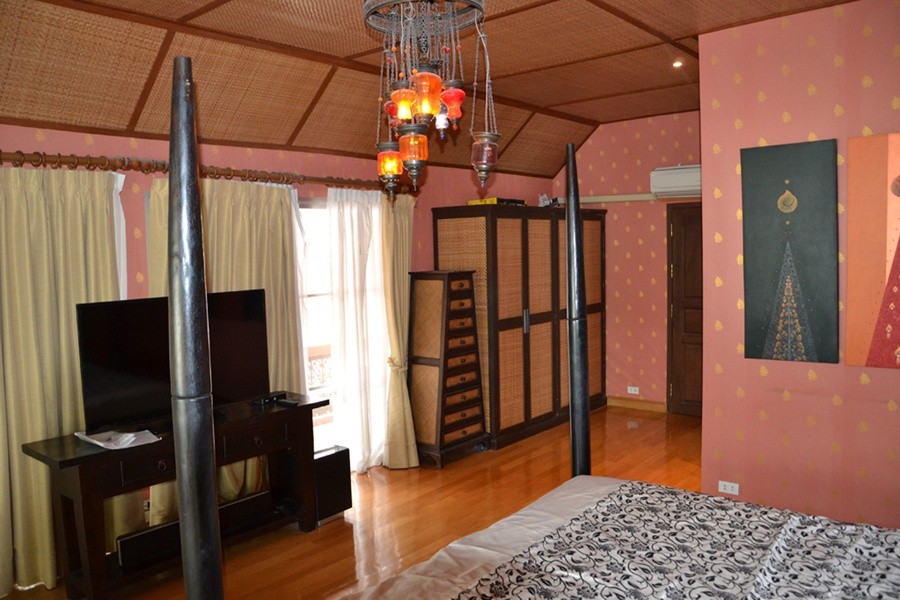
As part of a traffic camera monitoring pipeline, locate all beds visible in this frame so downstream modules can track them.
[353,475,900,600]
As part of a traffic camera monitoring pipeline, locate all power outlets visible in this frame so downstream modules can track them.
[718,480,740,495]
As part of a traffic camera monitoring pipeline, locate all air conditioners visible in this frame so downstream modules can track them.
[650,164,701,200]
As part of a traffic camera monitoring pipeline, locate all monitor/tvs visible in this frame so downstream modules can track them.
[75,289,272,435]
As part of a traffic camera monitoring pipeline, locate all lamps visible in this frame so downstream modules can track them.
[361,0,501,206]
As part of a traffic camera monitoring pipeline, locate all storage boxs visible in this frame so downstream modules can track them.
[467,197,528,206]
[313,443,352,523]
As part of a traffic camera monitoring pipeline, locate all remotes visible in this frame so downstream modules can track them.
[276,399,299,406]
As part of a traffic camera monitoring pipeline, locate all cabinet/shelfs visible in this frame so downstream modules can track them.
[406,266,491,469]
[428,204,608,452]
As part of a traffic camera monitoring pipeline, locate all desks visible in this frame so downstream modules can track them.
[20,391,331,600]
